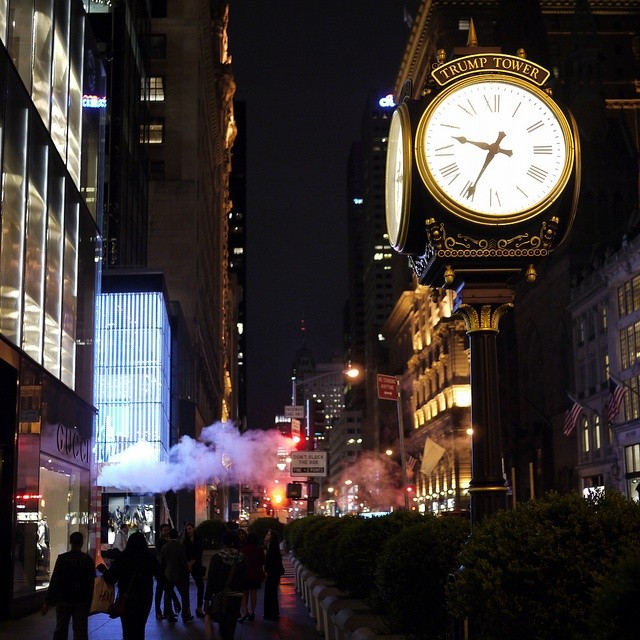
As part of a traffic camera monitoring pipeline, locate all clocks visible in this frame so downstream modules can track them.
[413,77,572,222]
[382,106,413,254]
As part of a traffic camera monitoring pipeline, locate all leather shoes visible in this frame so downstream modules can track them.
[183,616,194,623]
[167,615,177,622]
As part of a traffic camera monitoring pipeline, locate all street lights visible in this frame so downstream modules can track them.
[291,367,359,408]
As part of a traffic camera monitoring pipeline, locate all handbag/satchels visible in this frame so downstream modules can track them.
[210,589,231,622]
[90,576,114,614]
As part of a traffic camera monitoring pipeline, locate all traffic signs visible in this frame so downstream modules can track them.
[377,373,399,401]
[290,451,330,478]
[284,405,304,418]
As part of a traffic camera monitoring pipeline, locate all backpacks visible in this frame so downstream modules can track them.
[110,592,128,619]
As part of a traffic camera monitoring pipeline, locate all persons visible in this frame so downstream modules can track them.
[236,528,247,551]
[262,528,285,621]
[40,532,96,640]
[238,532,266,624]
[159,529,195,623]
[35,511,50,586]
[132,504,154,544]
[155,524,178,620]
[109,504,132,532]
[204,532,250,640]
[177,521,207,618]
[97,532,181,640]
[111,524,129,552]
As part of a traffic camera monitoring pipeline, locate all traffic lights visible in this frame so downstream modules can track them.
[262,502,270,508]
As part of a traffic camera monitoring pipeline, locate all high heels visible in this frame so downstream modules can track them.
[239,612,250,623]
[204,603,210,614]
[248,613,255,621]
[196,609,205,617]
